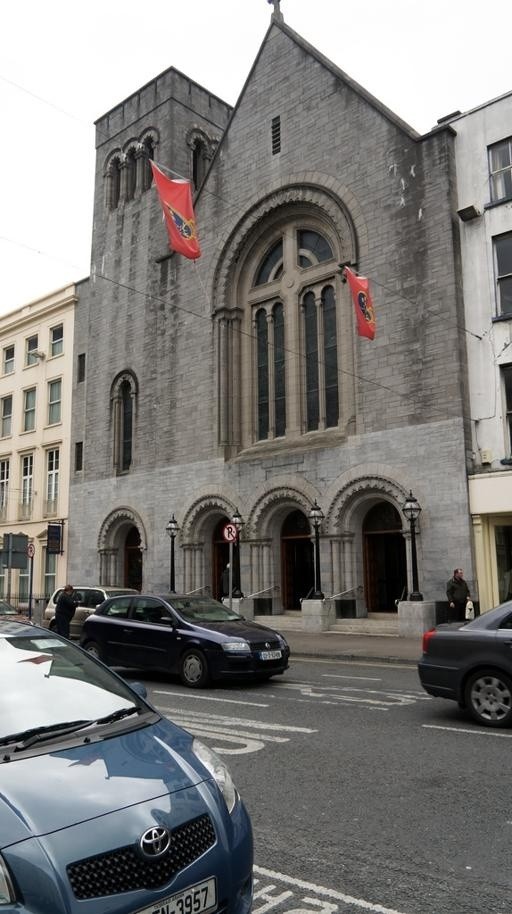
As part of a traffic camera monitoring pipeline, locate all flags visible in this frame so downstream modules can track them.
[149,159,201,259]
[345,266,376,340]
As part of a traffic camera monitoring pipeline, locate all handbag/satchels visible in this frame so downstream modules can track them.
[465,600,474,620]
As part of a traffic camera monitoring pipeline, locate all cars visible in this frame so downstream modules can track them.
[416,600,512,728]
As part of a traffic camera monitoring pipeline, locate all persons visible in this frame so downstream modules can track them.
[55,585,79,639]
[446,568,470,623]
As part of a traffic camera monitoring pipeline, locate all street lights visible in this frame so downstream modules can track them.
[308,498,325,599]
[165,513,181,594]
[230,506,245,598]
[402,489,424,601]
